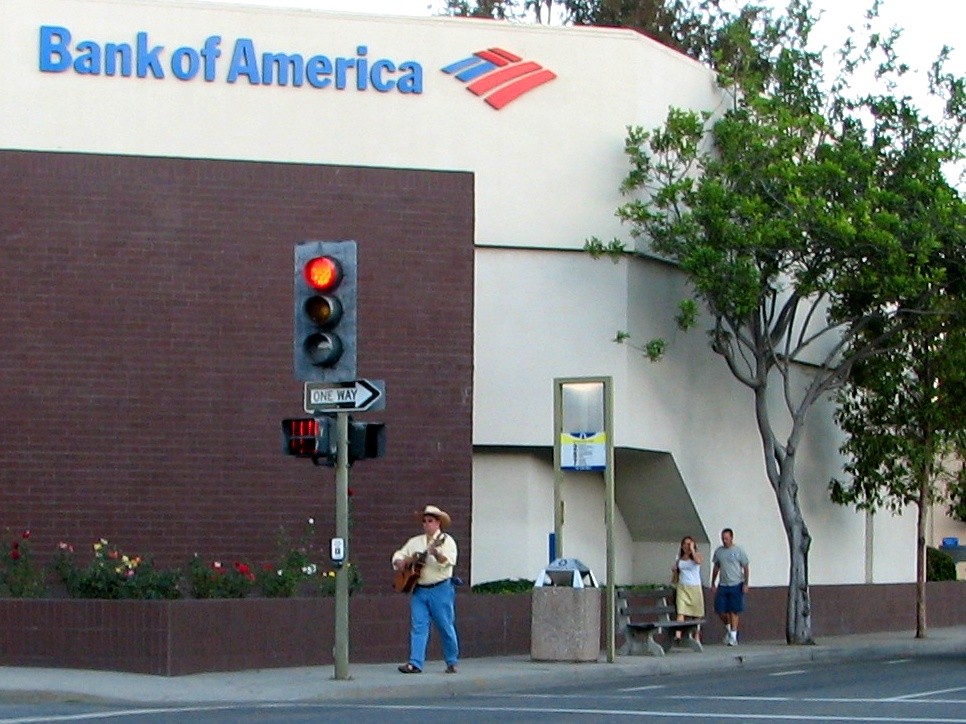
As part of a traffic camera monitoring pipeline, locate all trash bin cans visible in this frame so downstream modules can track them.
[530,559,600,662]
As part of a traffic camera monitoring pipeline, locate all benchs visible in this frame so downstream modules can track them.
[615,587,704,656]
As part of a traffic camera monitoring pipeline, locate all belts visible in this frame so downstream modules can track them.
[417,579,447,588]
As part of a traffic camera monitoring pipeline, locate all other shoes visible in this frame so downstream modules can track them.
[723,632,729,644]
[399,663,421,673]
[445,665,457,673]
[728,639,738,646]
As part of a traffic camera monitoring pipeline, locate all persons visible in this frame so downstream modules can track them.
[392,506,458,673]
[672,537,705,641]
[711,528,749,645]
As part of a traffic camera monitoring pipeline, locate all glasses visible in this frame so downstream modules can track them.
[422,519,434,522]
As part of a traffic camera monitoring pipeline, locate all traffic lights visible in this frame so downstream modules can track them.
[279,414,338,460]
[292,239,359,384]
[348,420,385,457]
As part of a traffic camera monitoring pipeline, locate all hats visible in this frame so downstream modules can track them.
[411,505,452,529]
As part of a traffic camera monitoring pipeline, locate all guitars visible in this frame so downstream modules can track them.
[391,537,446,593]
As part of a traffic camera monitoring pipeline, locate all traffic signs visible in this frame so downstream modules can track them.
[304,379,380,414]
